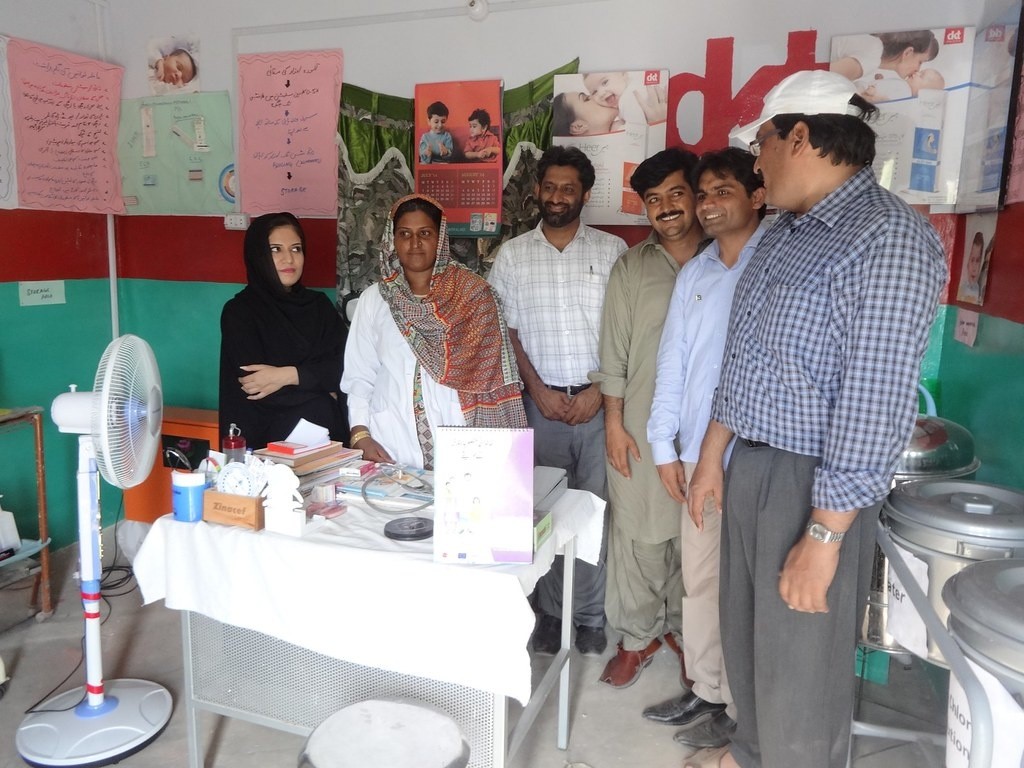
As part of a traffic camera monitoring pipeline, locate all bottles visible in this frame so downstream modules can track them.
[222,435,246,463]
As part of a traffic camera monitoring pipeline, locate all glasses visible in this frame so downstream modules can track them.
[748,129,781,157]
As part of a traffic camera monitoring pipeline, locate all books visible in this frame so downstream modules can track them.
[255,419,424,496]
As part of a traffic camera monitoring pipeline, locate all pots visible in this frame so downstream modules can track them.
[869,418,982,591]
[941,557,1024,767]
[880,479,1024,669]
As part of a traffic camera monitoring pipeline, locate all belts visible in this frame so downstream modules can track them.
[738,436,770,448]
[546,383,592,395]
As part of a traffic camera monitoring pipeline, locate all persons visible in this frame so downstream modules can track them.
[146,43,196,86]
[218,212,350,451]
[420,100,454,164]
[488,147,780,750]
[346,191,527,472]
[554,70,667,136]
[957,231,994,305]
[682,68,947,768]
[464,108,499,163]
[830,30,946,104]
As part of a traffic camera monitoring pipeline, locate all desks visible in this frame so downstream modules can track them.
[131,491,606,768]
[159,406,219,453]
[0,407,54,623]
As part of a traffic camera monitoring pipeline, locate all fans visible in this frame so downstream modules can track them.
[14,332,175,768]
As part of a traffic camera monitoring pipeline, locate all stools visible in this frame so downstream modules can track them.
[298,695,471,768]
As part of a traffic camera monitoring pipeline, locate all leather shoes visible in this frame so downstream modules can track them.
[575,625,607,657]
[643,691,728,725]
[673,710,738,749]
[532,616,563,656]
[665,633,696,690]
[599,637,662,688]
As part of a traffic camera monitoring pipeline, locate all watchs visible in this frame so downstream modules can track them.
[806,518,845,543]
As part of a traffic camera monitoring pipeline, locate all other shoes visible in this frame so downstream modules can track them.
[683,745,743,768]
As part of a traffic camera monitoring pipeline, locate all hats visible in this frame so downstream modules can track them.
[729,70,866,147]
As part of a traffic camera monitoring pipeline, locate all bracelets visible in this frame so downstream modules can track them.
[350,430,371,449]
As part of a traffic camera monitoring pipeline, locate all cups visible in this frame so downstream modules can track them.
[171,483,211,522]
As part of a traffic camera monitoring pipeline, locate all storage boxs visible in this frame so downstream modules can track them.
[204,486,267,528]
[534,509,553,550]
